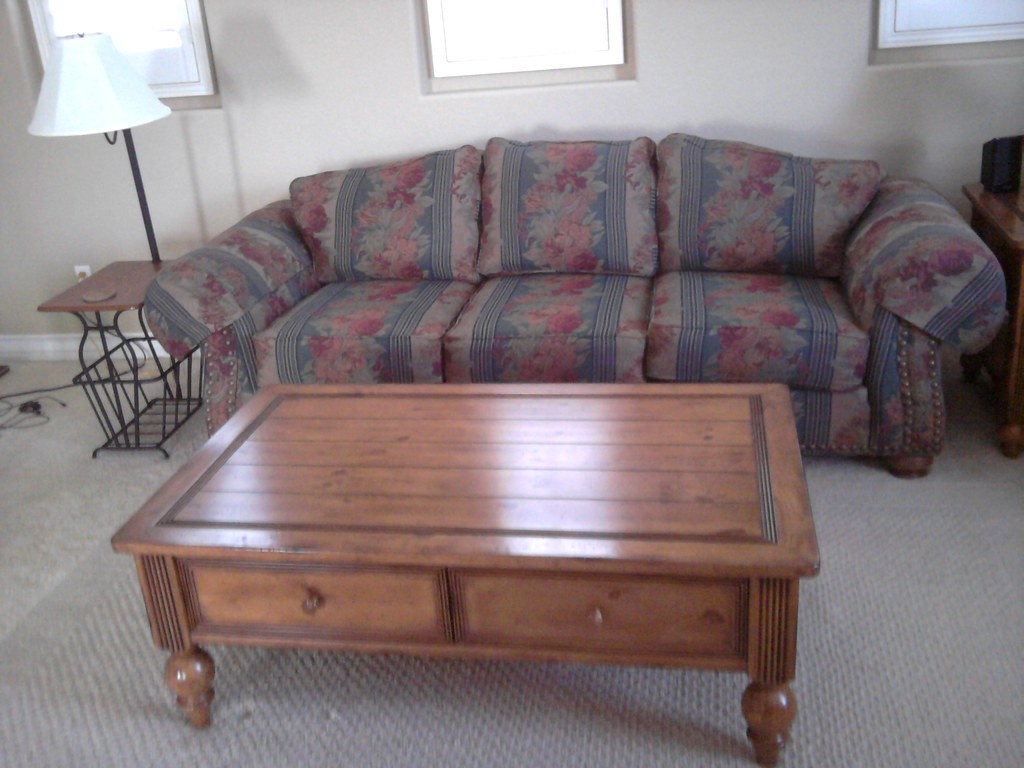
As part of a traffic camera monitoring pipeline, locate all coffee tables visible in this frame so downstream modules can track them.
[37,260,204,458]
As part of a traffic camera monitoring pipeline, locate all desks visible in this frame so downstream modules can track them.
[110,384,821,768]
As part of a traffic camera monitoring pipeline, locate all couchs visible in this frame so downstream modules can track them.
[144,133,1007,479]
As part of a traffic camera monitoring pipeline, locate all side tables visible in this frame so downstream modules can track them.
[956,183,1023,458]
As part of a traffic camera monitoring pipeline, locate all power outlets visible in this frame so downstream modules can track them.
[74,265,92,282]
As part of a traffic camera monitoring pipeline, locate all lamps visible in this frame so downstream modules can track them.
[27,33,171,261]
